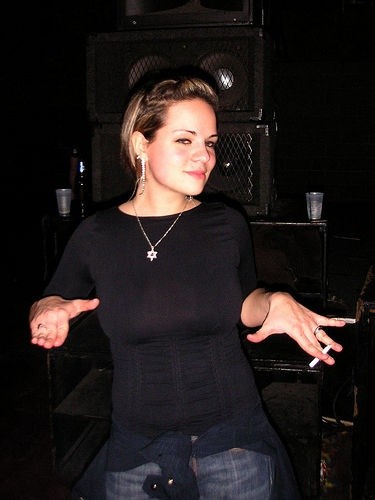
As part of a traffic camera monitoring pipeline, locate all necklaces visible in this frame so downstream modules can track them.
[126,192,187,261]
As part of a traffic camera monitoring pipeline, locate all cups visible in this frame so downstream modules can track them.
[56,188,72,215]
[306,192,323,222]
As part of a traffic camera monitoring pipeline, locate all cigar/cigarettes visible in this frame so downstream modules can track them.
[308,344,333,368]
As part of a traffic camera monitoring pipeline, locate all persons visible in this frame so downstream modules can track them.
[30,80,347,500]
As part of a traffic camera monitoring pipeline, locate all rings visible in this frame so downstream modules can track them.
[315,328,321,336]
[38,324,47,329]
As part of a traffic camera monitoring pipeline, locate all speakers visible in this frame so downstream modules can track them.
[86,0,272,221]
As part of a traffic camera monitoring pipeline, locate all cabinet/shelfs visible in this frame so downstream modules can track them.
[38,213,331,500]
